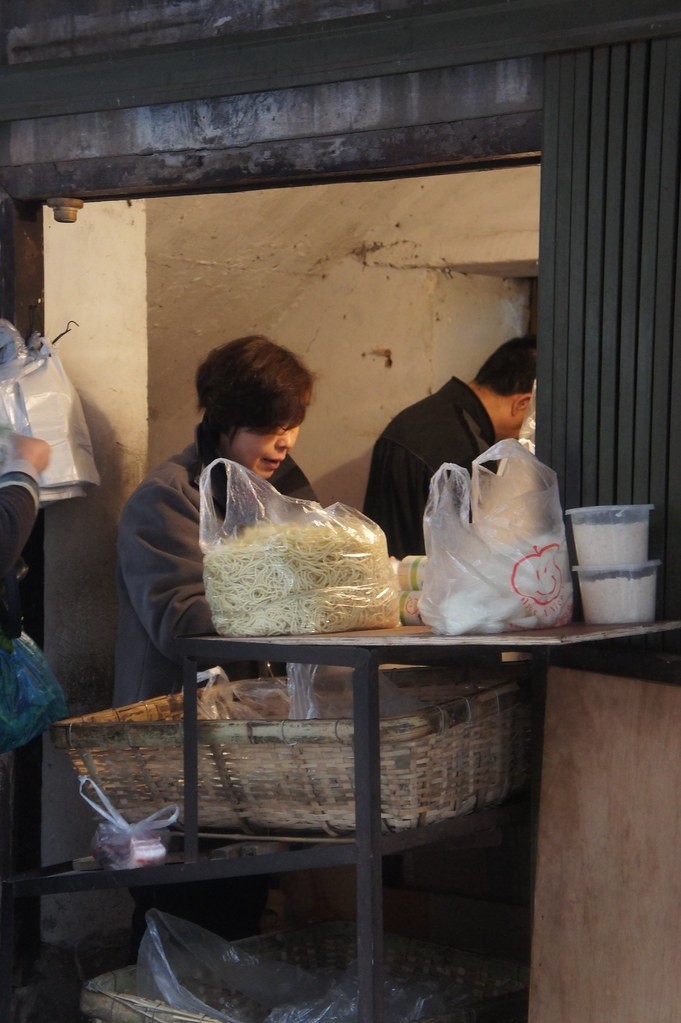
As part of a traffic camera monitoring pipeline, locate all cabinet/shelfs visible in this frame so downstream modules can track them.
[0,618,680,1022]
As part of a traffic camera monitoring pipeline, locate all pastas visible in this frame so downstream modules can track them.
[202,520,403,637]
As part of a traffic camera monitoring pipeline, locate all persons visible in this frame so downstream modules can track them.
[0,433,50,580]
[112,335,313,966]
[362,334,537,560]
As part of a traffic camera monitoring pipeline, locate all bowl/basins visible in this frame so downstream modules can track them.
[563,503,661,624]
